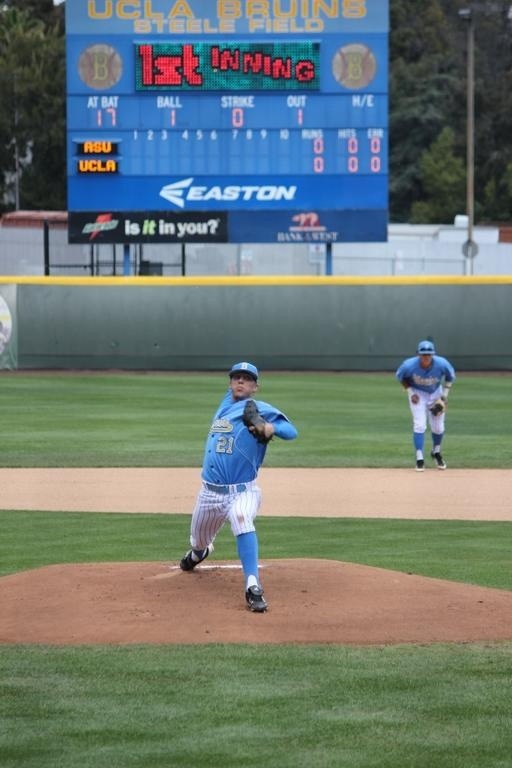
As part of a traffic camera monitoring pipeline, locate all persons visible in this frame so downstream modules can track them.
[394,339,454,472]
[179,361,298,612]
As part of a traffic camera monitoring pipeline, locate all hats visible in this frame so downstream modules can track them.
[228,363,258,379]
[417,341,435,355]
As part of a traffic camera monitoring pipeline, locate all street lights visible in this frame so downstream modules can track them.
[456,1,480,276]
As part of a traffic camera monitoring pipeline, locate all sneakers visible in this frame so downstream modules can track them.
[179,547,209,571]
[245,584,267,612]
[431,450,446,469]
[416,459,424,472]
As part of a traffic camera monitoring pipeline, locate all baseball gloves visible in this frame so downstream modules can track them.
[243,401,273,447]
[429,396,448,417]
